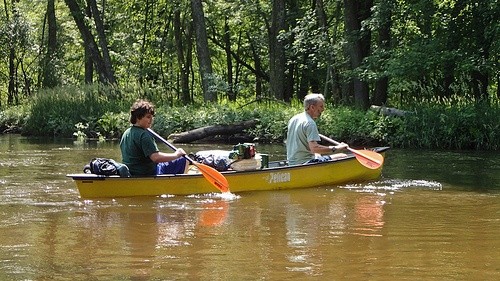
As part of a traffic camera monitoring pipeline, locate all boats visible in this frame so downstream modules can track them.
[67,144,390,199]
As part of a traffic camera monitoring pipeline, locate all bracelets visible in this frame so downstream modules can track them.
[330,146,337,153]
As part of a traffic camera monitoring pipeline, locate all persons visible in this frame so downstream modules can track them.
[286,93,349,167]
[120,99,190,175]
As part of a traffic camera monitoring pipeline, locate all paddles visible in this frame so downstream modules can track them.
[319,133,384,170]
[147,126,229,193]
